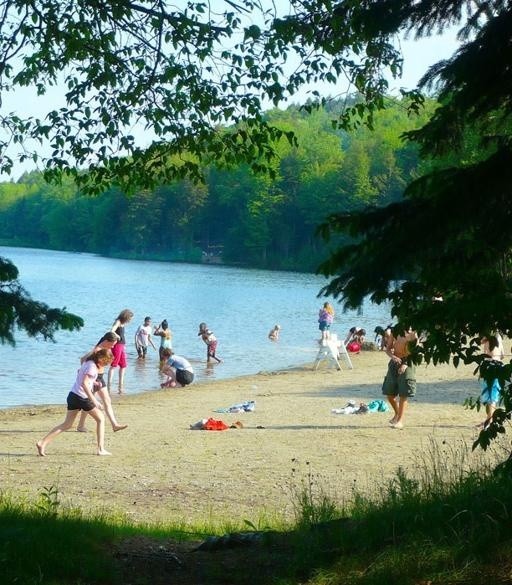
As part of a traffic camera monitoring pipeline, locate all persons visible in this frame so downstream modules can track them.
[161,348,196,387]
[374,326,394,350]
[198,323,222,362]
[344,326,367,349]
[77,332,128,432]
[154,319,171,369]
[269,324,280,340]
[476,328,503,428]
[382,326,419,429]
[36,348,112,456]
[135,317,156,360]
[319,301,334,344]
[108,309,134,394]
